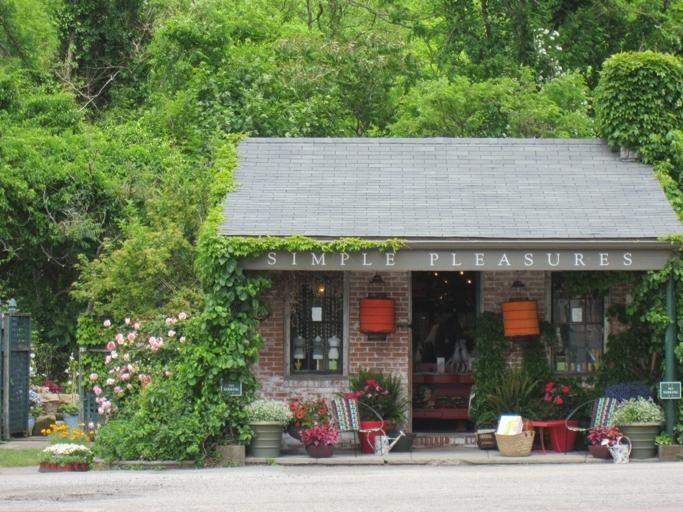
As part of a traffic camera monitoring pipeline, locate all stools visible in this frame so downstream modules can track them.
[523,420,558,454]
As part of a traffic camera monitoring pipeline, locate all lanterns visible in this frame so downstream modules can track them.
[356,291,396,342]
[500,296,541,344]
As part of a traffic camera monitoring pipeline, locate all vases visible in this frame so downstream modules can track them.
[304,442,333,457]
[358,421,389,453]
[27,413,34,436]
[550,419,579,452]
[587,443,611,457]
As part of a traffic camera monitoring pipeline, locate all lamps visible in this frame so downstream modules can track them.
[356,271,397,343]
[500,275,540,339]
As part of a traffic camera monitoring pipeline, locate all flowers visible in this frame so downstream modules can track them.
[346,365,406,424]
[287,397,337,445]
[38,421,95,473]
[584,425,622,446]
[27,390,45,416]
[543,375,584,422]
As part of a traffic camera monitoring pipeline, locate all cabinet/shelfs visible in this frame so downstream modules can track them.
[411,373,475,431]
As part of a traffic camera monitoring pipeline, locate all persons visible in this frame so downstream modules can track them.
[291,333,307,361]
[327,334,342,360]
[311,334,325,362]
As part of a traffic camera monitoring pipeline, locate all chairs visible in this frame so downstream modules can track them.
[328,396,361,456]
[557,395,622,458]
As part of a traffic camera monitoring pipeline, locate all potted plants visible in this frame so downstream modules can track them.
[613,392,665,461]
[654,424,682,462]
[57,401,78,426]
[240,398,292,459]
